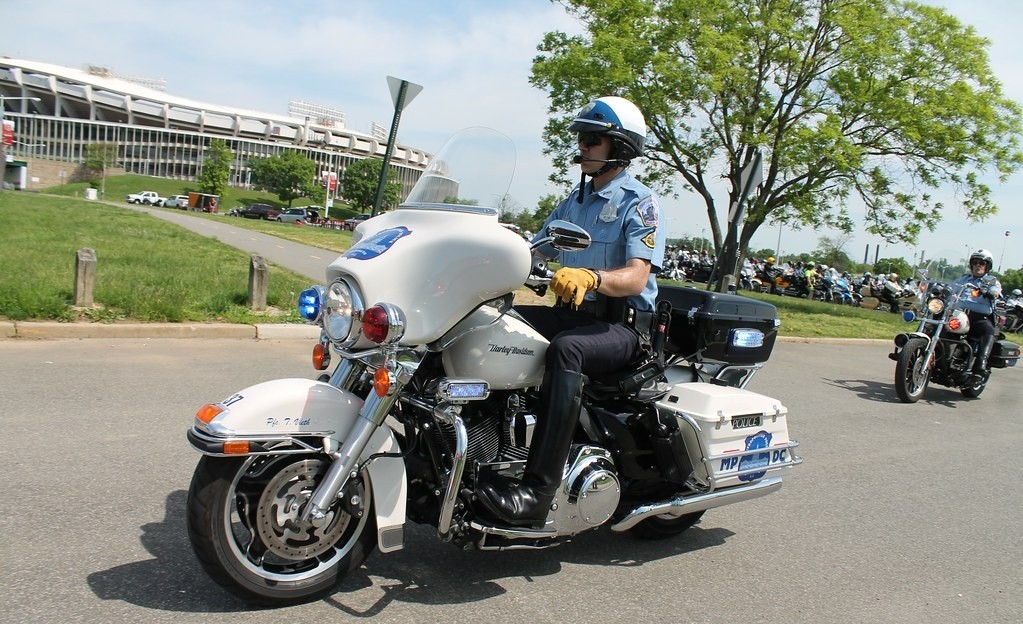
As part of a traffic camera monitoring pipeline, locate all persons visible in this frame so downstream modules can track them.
[296,211,356,230]
[919,249,1002,386]
[662,244,1023,333]
[463,95,666,528]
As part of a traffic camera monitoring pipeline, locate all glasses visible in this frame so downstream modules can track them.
[578,133,601,146]
[971,260,986,266]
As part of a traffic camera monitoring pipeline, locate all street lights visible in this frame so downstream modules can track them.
[0,94,41,188]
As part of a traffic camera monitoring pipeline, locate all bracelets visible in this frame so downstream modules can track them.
[586,267,602,291]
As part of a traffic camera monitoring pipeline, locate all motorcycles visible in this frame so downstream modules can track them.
[657,248,1023,335]
[887,249,1020,405]
[183,123,804,611]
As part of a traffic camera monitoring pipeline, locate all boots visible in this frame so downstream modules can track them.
[974,335,995,374]
[889,352,900,360]
[478,369,589,531]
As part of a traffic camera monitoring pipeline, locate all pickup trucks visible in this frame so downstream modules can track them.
[126,191,168,205]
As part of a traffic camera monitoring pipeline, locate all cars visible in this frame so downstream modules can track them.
[276,205,326,228]
[349,213,372,231]
[241,203,280,221]
[163,195,190,210]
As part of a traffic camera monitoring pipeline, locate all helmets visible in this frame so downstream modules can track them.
[767,256,775,265]
[691,250,699,254]
[750,257,759,263]
[682,245,688,248]
[665,245,678,249]
[1012,289,1022,296]
[787,260,852,278]
[970,250,993,272]
[862,272,897,281]
[701,250,708,255]
[907,278,914,283]
[566,96,646,158]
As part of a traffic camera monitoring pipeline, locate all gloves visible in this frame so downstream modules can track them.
[986,290,999,300]
[550,268,597,305]
